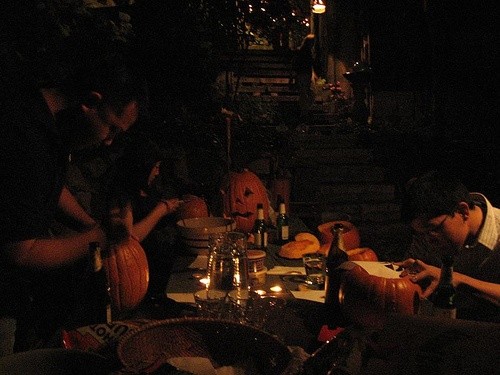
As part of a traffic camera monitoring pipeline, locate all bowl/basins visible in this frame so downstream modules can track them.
[246,250,266,273]
[177,218,237,251]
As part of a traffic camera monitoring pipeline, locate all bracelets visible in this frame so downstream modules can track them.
[160,199,170,212]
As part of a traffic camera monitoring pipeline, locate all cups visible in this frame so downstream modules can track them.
[205,232,249,294]
[302,255,326,285]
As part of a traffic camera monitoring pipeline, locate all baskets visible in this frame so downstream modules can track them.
[118,317,295,375]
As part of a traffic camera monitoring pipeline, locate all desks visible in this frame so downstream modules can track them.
[161,216,352,363]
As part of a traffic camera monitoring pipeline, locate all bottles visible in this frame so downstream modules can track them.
[324,224,348,306]
[252,203,269,251]
[273,198,291,243]
[85,241,112,325]
[435,255,458,320]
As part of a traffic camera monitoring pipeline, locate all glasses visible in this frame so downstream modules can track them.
[412,213,453,242]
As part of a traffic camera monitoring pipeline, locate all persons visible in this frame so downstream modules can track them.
[289,35,315,133]
[393,171,500,322]
[0,86,184,354]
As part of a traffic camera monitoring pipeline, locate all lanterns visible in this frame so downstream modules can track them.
[346,248,378,261]
[211,167,269,233]
[325,261,423,329]
[174,194,208,221]
[101,235,149,313]
[316,220,360,251]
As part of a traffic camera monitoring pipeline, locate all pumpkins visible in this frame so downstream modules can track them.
[102,237,150,311]
[177,195,207,219]
[277,220,424,317]
